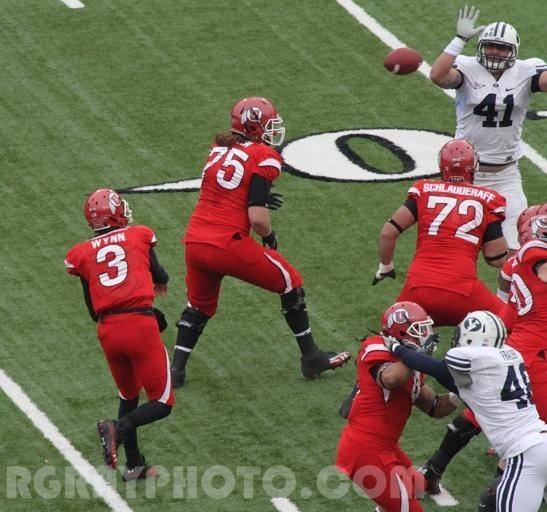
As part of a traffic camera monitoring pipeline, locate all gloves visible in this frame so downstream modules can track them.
[372,260,397,287]
[267,191,284,210]
[456,5,485,40]
[382,330,401,354]
[262,230,279,251]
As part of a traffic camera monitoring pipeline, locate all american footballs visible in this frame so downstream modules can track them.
[383,47,423,76]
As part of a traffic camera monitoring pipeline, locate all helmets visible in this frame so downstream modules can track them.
[229,95,287,148]
[437,137,482,185]
[516,204,547,246]
[451,310,506,351]
[379,301,439,357]
[476,21,520,75]
[83,186,134,234]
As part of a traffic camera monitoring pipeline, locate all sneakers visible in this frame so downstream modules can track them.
[120,461,164,482]
[414,466,442,496]
[338,381,361,418]
[96,419,123,470]
[300,350,351,381]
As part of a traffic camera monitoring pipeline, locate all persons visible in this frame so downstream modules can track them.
[170,96,349,392]
[64,186,175,479]
[381,309,547,511]
[478,203,547,509]
[421,204,546,507]
[337,141,509,425]
[429,5,547,304]
[335,300,461,512]
